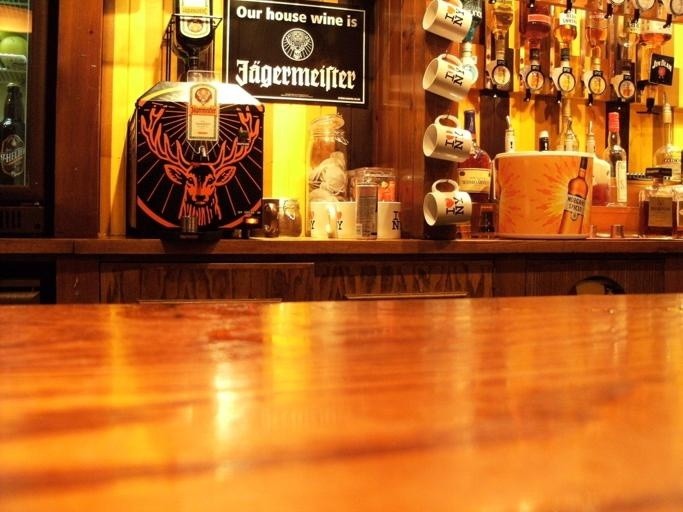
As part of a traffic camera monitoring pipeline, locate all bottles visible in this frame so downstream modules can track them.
[537,131,550,151]
[460,0,674,115]
[458,110,493,201]
[0,82,25,185]
[306,113,396,240]
[582,103,682,237]
[170,0,214,83]
[559,157,588,233]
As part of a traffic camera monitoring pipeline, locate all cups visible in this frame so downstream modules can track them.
[477,205,496,238]
[423,0,473,228]
[377,201,402,240]
